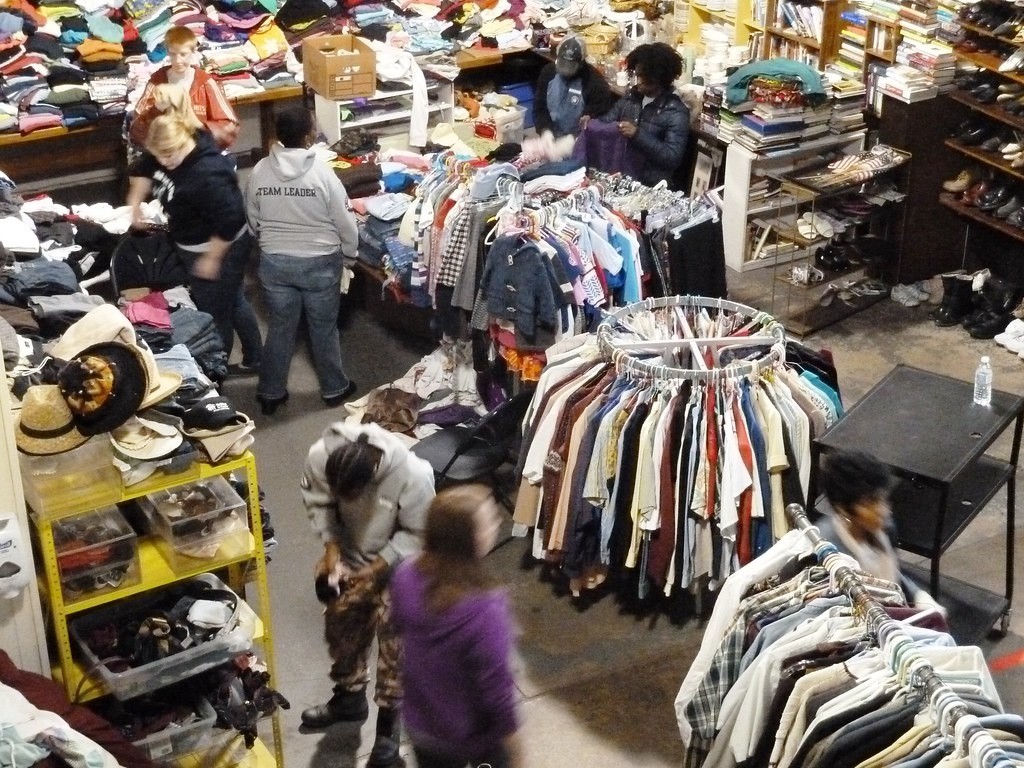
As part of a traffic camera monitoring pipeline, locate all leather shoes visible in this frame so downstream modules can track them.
[941,165,1024,229]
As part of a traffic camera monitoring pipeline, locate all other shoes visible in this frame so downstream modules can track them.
[261,387,289,415]
[963,3,1024,71]
[951,116,1024,168]
[956,71,1024,115]
[325,379,356,406]
[786,232,898,307]
[224,361,261,377]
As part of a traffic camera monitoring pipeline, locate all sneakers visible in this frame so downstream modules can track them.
[889,269,1024,360]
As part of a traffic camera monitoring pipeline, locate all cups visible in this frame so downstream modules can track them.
[617,72,629,86]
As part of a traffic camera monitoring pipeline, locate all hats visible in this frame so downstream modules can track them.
[106,414,183,459]
[554,36,586,74]
[58,341,149,436]
[182,396,247,430]
[134,348,184,411]
[14,385,94,455]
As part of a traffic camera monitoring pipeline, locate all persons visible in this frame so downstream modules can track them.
[533,34,611,145]
[128,115,263,377]
[245,107,359,415]
[128,27,239,174]
[579,39,694,238]
[301,424,437,768]
[811,450,944,628]
[391,485,526,768]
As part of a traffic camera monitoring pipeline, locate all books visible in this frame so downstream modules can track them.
[700,0,967,153]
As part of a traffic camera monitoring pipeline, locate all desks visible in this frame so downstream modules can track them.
[0,82,304,206]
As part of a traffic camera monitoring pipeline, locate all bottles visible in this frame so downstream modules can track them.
[973,356,992,405]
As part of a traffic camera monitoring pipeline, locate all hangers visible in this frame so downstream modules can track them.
[415,148,1024,768]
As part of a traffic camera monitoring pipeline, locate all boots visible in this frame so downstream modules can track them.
[371,707,401,765]
[301,685,369,727]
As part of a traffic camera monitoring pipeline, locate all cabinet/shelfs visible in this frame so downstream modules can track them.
[808,359,1024,648]
[314,78,456,153]
[687,0,1024,344]
[23,448,283,768]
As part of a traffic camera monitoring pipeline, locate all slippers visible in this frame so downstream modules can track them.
[794,210,847,240]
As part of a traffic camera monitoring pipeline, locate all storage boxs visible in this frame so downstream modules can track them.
[69,572,259,702]
[131,693,218,760]
[301,35,377,99]
[495,105,527,144]
[496,81,536,129]
